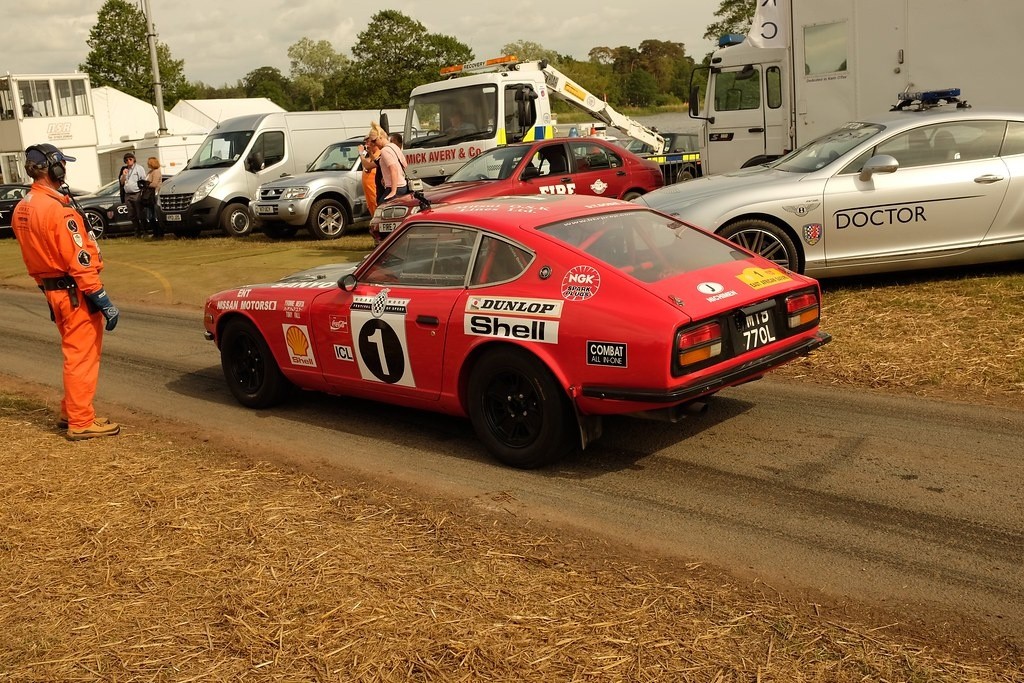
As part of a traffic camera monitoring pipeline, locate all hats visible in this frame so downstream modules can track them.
[27,144,76,168]
[363,135,369,141]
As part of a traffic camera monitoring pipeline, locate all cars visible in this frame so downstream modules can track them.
[200,197,838,462]
[247,130,403,238]
[617,107,1024,283]
[70,175,176,242]
[369,133,664,254]
[154,110,437,233]
[0,182,95,238]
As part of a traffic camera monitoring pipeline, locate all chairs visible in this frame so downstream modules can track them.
[907,129,958,160]
[549,153,568,172]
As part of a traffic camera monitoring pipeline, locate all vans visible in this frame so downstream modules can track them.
[688,0,1024,187]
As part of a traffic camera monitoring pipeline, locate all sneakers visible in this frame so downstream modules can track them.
[55,414,110,429]
[66,420,120,440]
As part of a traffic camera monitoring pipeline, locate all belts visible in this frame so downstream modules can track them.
[42,275,78,290]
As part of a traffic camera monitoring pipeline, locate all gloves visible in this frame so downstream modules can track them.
[89,286,118,331]
[38,285,56,323]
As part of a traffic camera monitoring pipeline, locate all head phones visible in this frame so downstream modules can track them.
[25,144,66,182]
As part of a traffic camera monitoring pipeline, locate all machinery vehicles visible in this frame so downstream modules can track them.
[400,55,703,186]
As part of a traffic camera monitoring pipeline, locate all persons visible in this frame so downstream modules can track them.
[118,153,164,242]
[444,111,478,136]
[12,144,120,440]
[356,121,411,248]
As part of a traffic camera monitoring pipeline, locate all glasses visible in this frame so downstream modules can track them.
[125,159,132,161]
[371,137,378,143]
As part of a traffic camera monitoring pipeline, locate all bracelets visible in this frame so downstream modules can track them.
[358,150,366,155]
